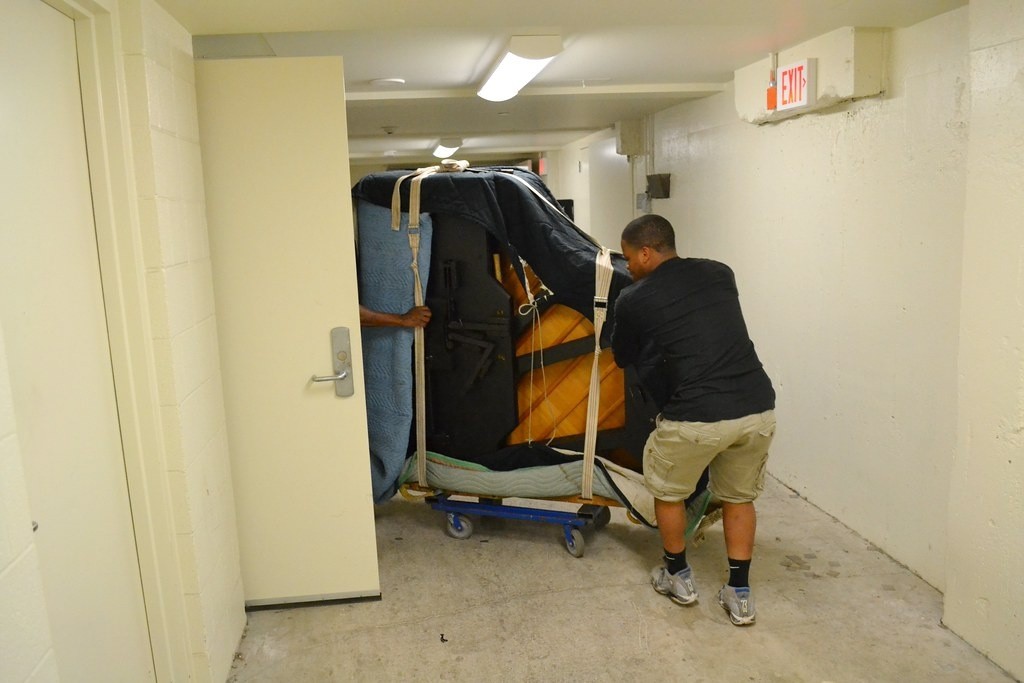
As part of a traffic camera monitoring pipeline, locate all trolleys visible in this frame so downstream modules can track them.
[405,478,612,559]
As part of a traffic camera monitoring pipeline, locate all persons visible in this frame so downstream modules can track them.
[610,214,776,625]
[359,303,432,327]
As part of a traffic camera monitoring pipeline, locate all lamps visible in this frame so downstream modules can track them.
[432,138,464,159]
[477,35,563,102]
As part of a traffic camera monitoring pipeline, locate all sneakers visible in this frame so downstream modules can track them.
[717,582,755,625]
[649,564,699,605]
